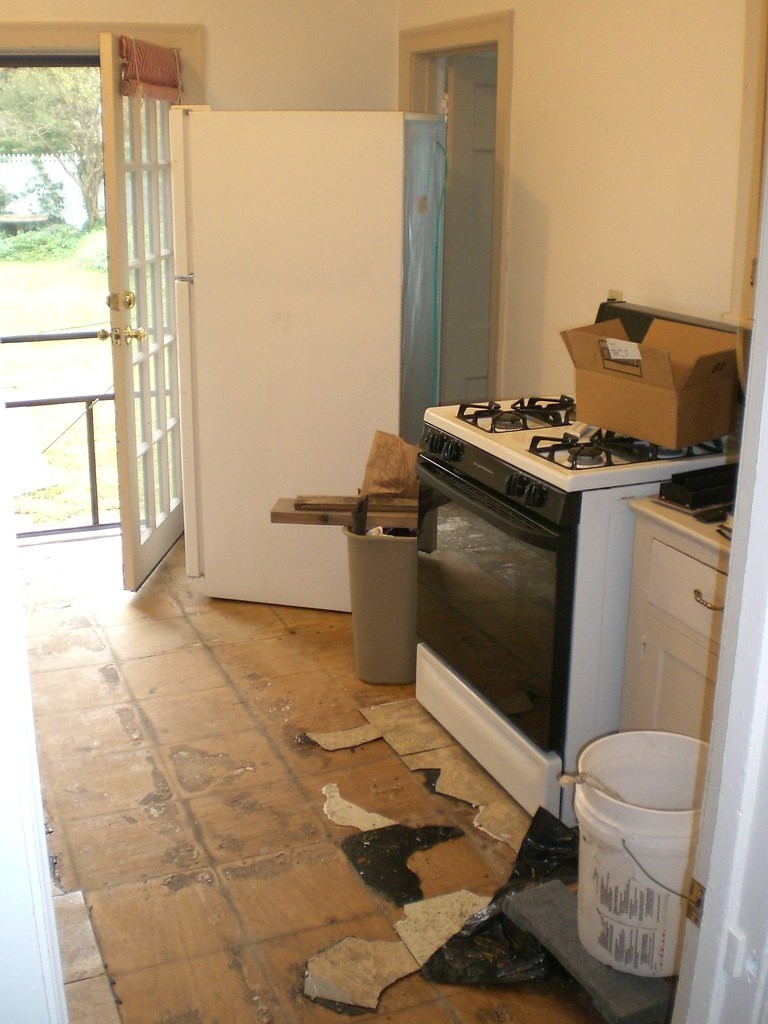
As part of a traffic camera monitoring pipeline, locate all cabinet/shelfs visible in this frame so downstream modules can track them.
[617,497,733,742]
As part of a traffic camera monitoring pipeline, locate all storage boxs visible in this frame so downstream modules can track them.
[561,318,741,449]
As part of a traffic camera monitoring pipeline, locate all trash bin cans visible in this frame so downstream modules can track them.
[342,525,419,685]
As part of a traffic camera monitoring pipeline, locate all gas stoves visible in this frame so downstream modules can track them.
[424,392,725,477]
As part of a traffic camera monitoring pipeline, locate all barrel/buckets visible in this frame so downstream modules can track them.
[573,730,710,979]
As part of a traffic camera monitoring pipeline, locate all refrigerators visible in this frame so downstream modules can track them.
[168,104,447,612]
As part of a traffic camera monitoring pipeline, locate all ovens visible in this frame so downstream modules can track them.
[415,424,581,752]
